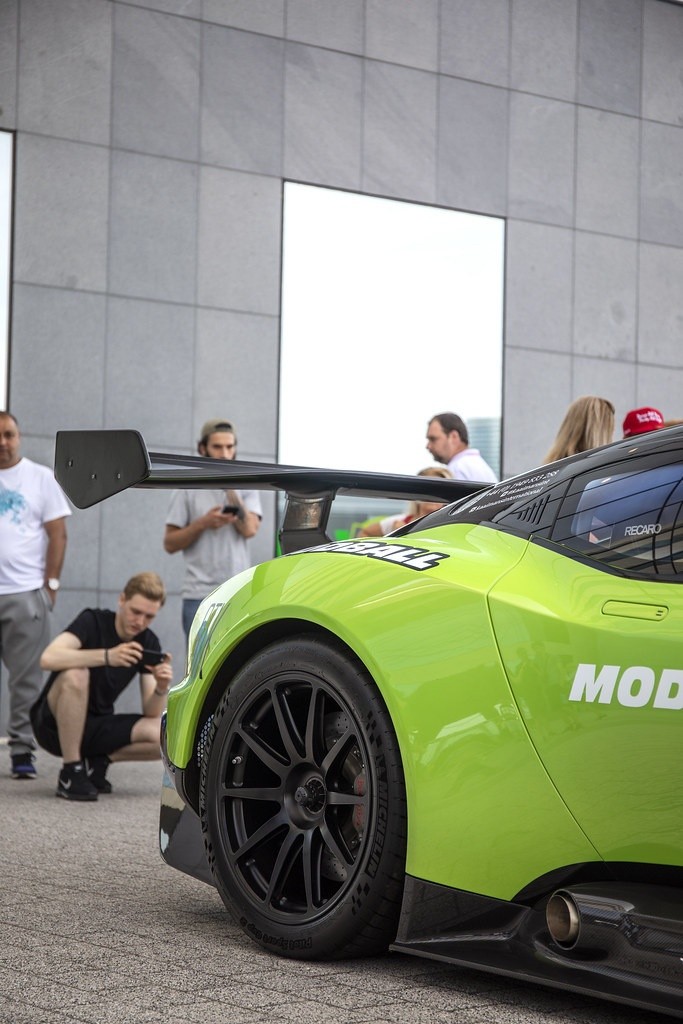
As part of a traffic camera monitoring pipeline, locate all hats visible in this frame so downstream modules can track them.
[201,418,235,437]
[623,407,665,437]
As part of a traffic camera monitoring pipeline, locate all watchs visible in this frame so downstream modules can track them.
[48,577,59,590]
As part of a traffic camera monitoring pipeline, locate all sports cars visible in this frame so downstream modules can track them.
[55,425,681,1021]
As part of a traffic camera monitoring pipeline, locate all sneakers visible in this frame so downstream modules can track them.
[56,762,98,800]
[82,754,112,793]
[10,753,37,779]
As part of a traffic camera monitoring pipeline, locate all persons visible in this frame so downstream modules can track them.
[29,572,173,800]
[425,413,499,483]
[544,396,616,464]
[364,468,456,536]
[622,406,663,438]
[0,412,73,778]
[165,419,264,652]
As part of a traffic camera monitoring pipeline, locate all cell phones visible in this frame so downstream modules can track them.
[139,649,167,666]
[223,505,239,515]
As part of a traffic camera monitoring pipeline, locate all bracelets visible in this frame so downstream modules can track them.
[155,689,169,695]
[105,647,109,665]
